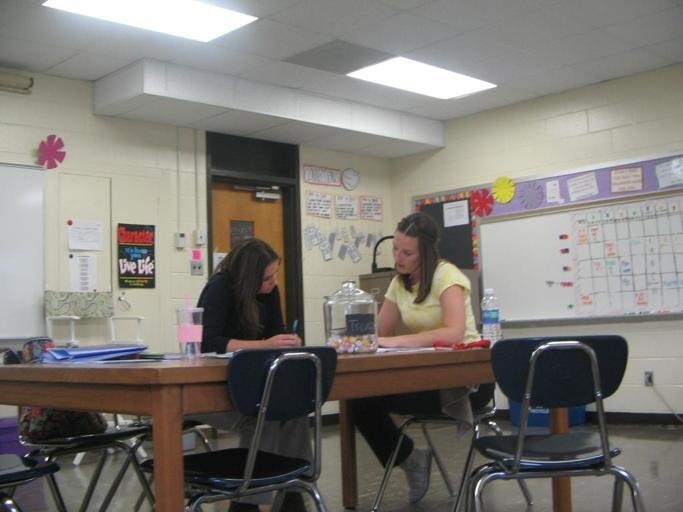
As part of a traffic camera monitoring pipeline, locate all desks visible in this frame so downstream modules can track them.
[0,347,573,512]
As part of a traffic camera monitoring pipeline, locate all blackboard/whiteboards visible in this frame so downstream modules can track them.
[0,160,49,343]
[479,187,683,327]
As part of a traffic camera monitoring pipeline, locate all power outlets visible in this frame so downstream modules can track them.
[645,372,653,386]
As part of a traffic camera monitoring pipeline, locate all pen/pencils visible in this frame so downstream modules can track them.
[292,320,297,334]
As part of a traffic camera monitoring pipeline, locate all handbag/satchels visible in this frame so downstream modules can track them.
[18,338,107,439]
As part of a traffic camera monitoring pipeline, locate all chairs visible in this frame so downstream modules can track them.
[466,336,643,512]
[371,383,532,512]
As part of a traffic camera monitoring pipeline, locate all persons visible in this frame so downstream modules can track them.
[178,238,316,511]
[345,213,495,508]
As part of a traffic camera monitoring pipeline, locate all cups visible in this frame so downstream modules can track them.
[175,307,204,362]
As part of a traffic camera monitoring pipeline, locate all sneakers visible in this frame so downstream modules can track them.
[281,491,307,511]
[407,446,432,504]
[228,501,260,512]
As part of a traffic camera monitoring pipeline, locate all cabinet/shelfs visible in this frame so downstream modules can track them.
[359,269,481,332]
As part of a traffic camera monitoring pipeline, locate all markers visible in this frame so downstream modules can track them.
[624,308,683,314]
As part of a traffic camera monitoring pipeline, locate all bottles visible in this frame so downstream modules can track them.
[322,281,378,355]
[480,288,501,348]
